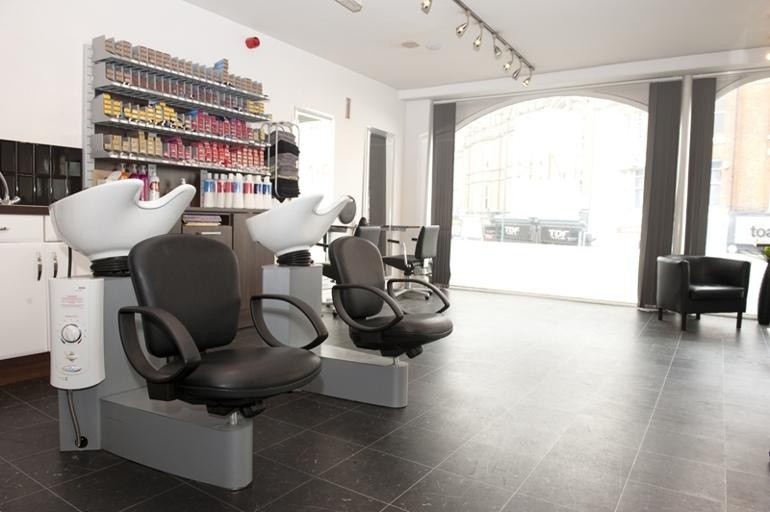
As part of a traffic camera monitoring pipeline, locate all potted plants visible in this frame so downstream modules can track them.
[757,246,770,326]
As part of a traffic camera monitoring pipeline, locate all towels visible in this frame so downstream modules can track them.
[270,130,300,197]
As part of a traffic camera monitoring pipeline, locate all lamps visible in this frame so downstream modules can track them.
[422,0,536,88]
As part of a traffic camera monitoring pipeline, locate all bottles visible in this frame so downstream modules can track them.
[120,161,160,202]
[204,171,274,210]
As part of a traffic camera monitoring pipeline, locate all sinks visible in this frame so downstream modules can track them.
[246,195,351,251]
[47,178,196,258]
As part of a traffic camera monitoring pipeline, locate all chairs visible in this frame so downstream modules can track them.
[320,226,385,319]
[327,235,452,370]
[383,225,442,303]
[656,256,751,331]
[116,231,331,424]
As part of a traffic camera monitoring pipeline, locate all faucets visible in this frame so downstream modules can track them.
[0,171,11,201]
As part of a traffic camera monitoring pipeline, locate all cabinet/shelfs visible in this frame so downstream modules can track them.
[0,139,83,215]
[0,242,94,360]
[91,35,272,331]
